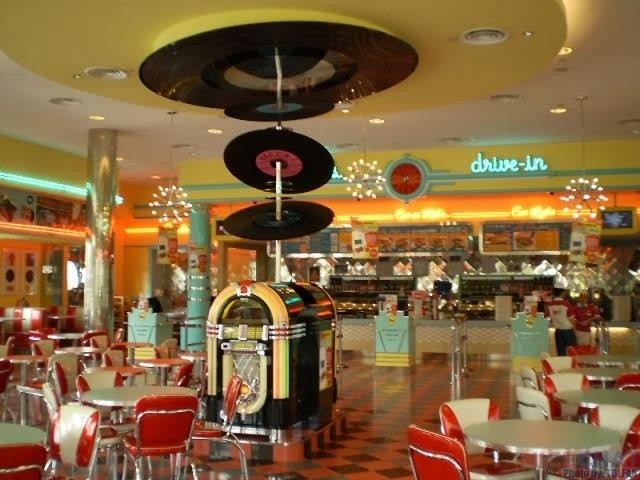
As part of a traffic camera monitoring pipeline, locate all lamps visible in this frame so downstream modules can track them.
[558,96,609,222]
[147,111,198,225]
[340,98,387,203]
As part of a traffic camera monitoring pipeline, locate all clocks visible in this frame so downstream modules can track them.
[384,152,430,205]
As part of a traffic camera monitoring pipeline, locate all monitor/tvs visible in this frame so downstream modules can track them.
[596,206,638,236]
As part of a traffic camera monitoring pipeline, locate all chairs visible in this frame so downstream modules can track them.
[1,358,11,393]
[15,352,78,426]
[75,368,127,411]
[0,303,249,388]
[1,441,47,480]
[405,343,639,480]
[122,394,198,479]
[45,404,101,480]
[73,375,122,480]
[40,382,61,414]
[182,375,249,480]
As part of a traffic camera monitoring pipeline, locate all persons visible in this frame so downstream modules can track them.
[544,288,601,356]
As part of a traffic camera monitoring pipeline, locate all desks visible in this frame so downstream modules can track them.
[84,384,199,476]
[2,423,46,445]
